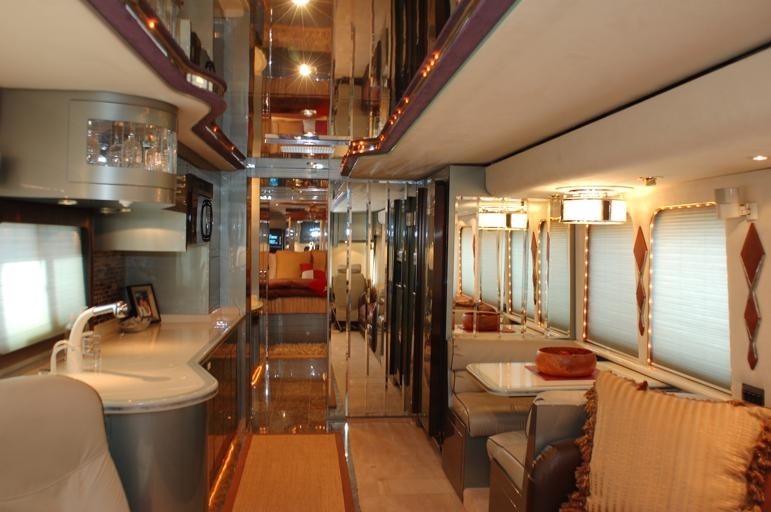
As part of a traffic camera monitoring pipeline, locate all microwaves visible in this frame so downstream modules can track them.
[190,197,216,250]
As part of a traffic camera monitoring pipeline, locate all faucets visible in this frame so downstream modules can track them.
[66,301,128,370]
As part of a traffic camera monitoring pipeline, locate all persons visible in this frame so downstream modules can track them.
[136,296,152,317]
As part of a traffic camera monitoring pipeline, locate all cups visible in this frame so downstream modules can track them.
[81,331,102,369]
[86,120,178,173]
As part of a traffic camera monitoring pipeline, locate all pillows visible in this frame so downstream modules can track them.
[557,370,771,511]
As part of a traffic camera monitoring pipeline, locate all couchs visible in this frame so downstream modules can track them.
[266,250,327,343]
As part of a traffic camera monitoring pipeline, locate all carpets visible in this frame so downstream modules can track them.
[268,343,327,358]
[348,417,468,512]
[220,434,355,512]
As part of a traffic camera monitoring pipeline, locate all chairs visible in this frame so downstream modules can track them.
[334,264,367,322]
[358,287,381,353]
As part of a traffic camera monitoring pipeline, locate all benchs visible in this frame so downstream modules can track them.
[485,391,719,510]
[442,340,580,503]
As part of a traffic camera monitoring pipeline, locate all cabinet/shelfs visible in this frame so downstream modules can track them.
[200,326,239,495]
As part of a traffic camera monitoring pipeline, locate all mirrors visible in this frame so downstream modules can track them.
[328,176,426,419]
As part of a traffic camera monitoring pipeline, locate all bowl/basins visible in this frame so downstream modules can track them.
[461,312,500,330]
[119,317,152,333]
[534,346,598,377]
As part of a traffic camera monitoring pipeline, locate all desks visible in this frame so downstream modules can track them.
[462,356,671,399]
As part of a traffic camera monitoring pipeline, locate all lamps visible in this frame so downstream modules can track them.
[561,186,634,225]
[714,188,758,221]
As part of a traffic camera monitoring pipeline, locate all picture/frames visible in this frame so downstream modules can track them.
[126,283,161,324]
